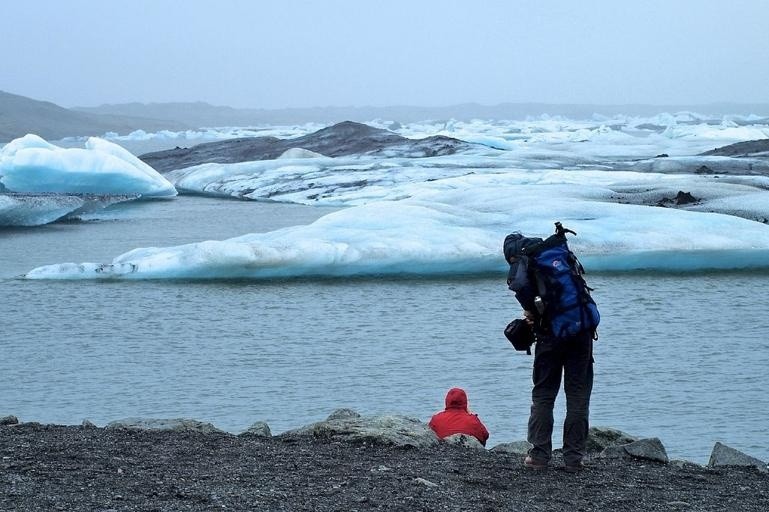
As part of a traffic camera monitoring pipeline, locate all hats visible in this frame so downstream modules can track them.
[503,235,525,259]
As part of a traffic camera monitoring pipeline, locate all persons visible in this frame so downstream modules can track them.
[429,387,489,448]
[503,229,600,472]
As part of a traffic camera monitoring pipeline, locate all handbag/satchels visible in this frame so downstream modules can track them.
[504,319,534,351]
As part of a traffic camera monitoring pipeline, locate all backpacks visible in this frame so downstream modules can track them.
[526,234,600,340]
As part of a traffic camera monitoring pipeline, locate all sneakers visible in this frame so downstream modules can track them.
[525,456,546,470]
[565,460,584,473]
